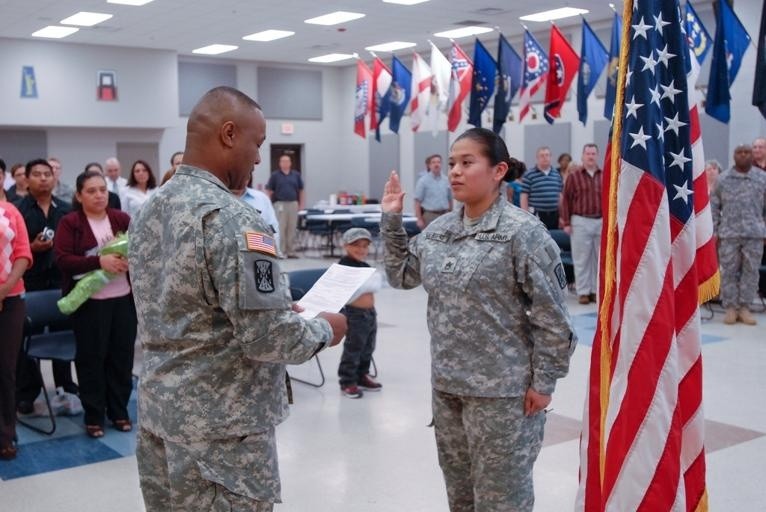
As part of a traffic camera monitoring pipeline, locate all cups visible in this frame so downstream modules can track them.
[329,194,337,205]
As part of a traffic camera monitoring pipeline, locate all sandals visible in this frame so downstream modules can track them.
[111,418,132,431]
[87,424,104,437]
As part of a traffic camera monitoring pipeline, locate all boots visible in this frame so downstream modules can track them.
[739,305,757,325]
[725,303,739,324]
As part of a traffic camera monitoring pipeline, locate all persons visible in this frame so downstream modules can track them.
[418,157,430,178]
[506,144,603,304]
[129,86,348,512]
[230,174,280,250]
[264,155,304,258]
[339,227,383,399]
[378,128,579,511]
[704,138,766,325]
[1,151,183,460]
[414,154,453,231]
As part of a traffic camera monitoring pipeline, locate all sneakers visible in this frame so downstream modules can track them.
[340,382,362,399]
[1,441,16,459]
[357,376,382,391]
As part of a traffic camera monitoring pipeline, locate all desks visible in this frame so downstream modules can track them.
[313,204,382,248]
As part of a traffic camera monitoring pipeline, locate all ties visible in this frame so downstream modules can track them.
[112,179,119,196]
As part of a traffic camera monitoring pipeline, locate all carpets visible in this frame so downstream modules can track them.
[0,373,138,481]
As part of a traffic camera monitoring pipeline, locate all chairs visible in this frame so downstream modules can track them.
[549,229,574,290]
[285,268,379,388]
[295,209,420,266]
[16,288,75,438]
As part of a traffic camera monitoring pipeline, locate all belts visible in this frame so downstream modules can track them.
[576,214,604,219]
[431,211,448,214]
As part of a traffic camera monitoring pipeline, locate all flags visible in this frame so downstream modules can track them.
[575,0,711,512]
[354,0,766,143]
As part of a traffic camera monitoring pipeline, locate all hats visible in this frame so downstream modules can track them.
[342,227,373,245]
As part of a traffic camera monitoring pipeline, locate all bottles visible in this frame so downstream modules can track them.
[339,193,367,206]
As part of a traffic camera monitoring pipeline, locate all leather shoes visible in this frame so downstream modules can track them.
[288,254,299,259]
[579,294,590,304]
[590,293,597,302]
